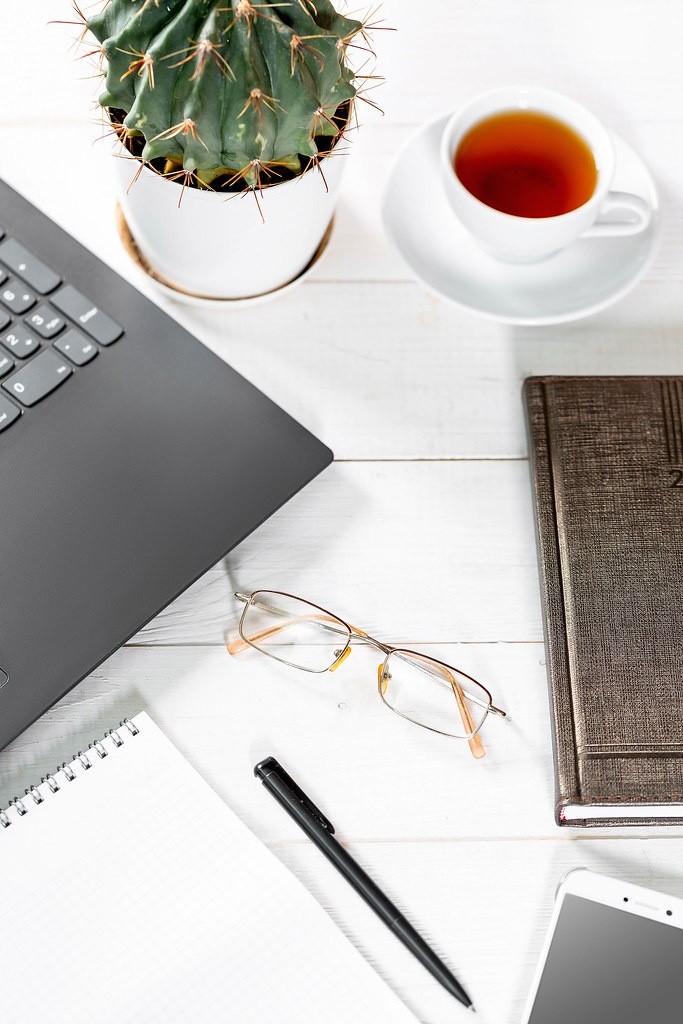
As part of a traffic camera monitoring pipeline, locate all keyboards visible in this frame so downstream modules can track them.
[0,180,335,755]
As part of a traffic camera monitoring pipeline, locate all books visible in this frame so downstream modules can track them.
[520,375,683,829]
[1,710,423,1024]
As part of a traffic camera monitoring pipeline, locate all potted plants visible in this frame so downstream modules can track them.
[46,0,399,310]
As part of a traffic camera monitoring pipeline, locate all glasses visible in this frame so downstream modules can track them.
[227,590,507,759]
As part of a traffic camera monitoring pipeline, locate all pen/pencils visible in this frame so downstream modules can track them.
[246,747,486,1017]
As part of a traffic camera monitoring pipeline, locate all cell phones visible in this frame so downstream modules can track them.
[520,865,683,1024]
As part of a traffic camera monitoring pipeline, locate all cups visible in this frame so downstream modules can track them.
[441,86,651,264]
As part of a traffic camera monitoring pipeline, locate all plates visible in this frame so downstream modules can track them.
[381,110,663,325]
